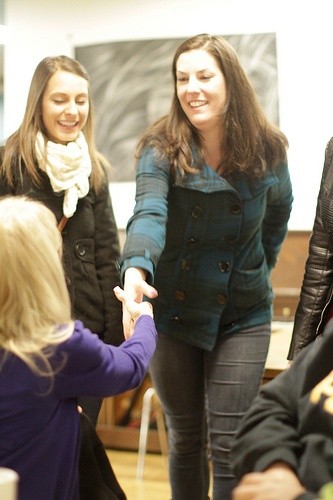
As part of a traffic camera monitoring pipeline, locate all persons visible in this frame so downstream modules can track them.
[0,196,158,500]
[0,55,125,429]
[287,136,333,364]
[227,318,333,500]
[118,32,294,500]
